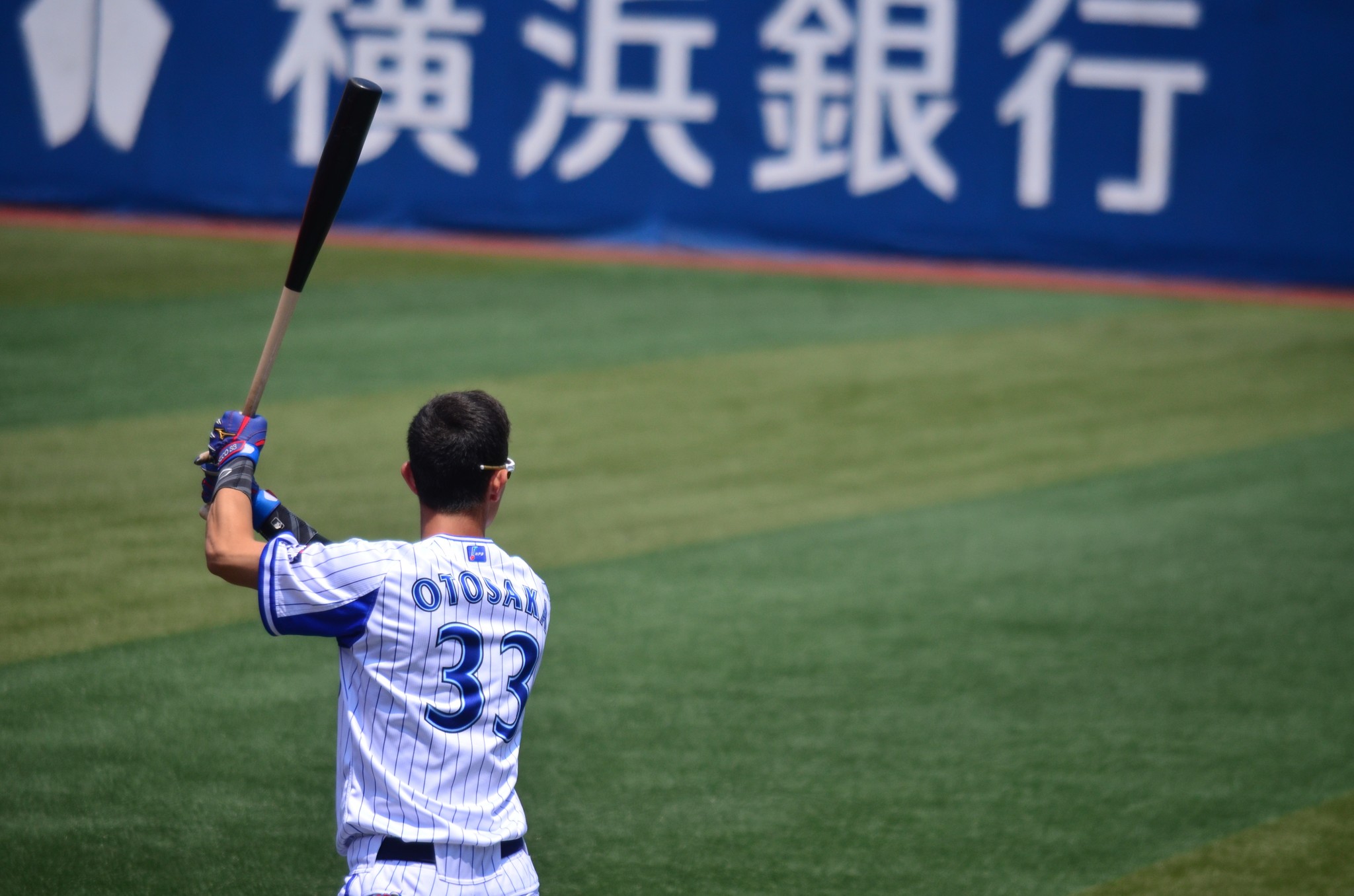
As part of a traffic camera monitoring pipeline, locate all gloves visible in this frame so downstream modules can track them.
[194,450,282,527]
[207,409,269,469]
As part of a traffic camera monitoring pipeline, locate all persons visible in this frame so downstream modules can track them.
[194,391,552,896]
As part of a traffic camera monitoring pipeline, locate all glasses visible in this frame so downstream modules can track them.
[480,456,515,477]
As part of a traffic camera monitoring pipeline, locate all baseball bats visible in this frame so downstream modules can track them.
[201,77,381,520]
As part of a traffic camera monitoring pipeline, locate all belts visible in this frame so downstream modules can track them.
[377,837,524,864]
